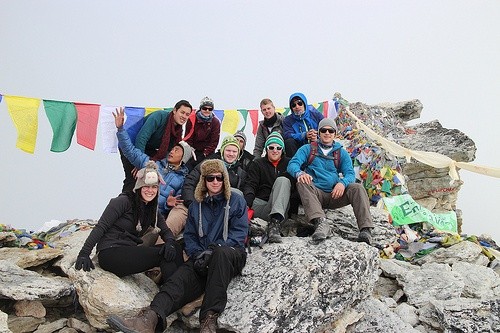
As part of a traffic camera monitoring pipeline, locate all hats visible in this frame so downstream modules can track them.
[199,96,214,110]
[232,131,247,142]
[194,159,230,242]
[264,131,285,151]
[318,118,337,139]
[132,160,166,234]
[172,140,197,170]
[219,135,240,163]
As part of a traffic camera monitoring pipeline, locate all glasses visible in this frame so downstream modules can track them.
[319,127,335,134]
[291,100,303,108]
[202,106,213,111]
[266,145,282,151]
[205,174,224,182]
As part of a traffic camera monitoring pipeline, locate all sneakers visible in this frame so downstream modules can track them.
[311,217,333,241]
[358,228,373,245]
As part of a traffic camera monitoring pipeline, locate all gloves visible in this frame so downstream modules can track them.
[75,250,96,272]
[158,238,177,262]
[193,247,213,272]
[243,193,256,211]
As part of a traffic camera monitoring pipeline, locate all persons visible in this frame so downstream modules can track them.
[112,107,196,212]
[243,131,301,247]
[75,160,185,285]
[184,96,221,158]
[286,118,376,246]
[117,100,193,193]
[182,132,256,208]
[106,159,248,333]
[253,98,297,161]
[282,92,324,158]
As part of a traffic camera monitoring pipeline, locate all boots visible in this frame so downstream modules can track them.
[268,218,282,243]
[106,306,158,333]
[199,313,219,333]
